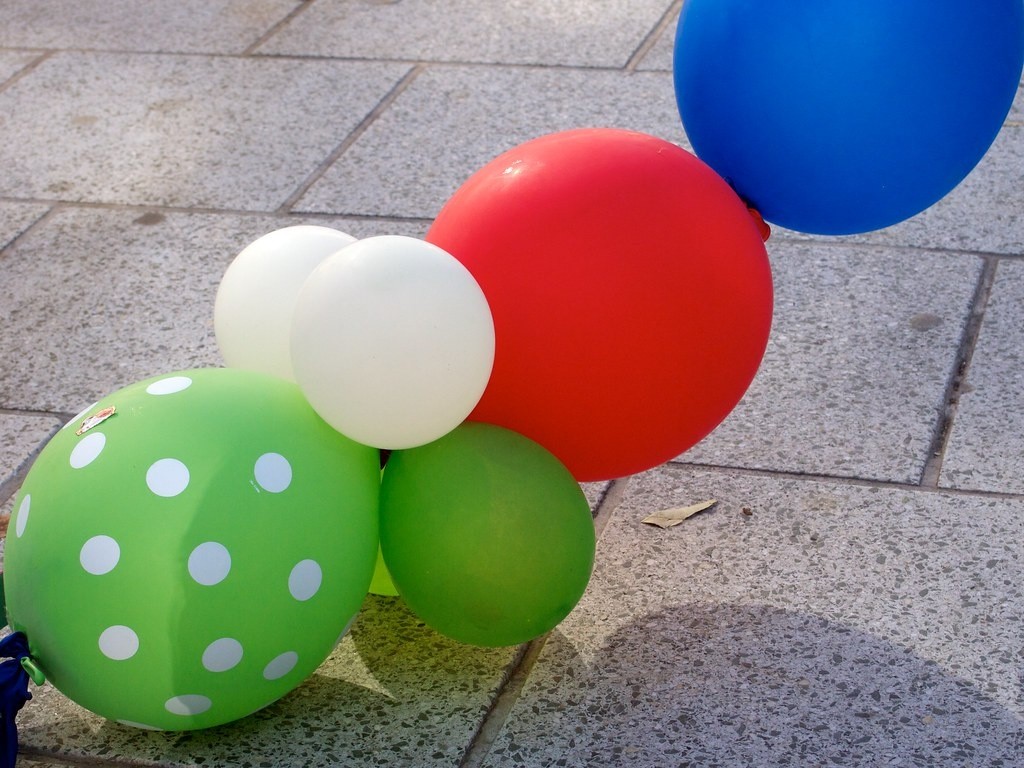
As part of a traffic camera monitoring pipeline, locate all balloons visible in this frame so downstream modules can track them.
[673,0,1024,236]
[290,235,496,450]
[3,368,381,732]
[425,128,774,482]
[366,421,596,648]
[213,226,359,373]
